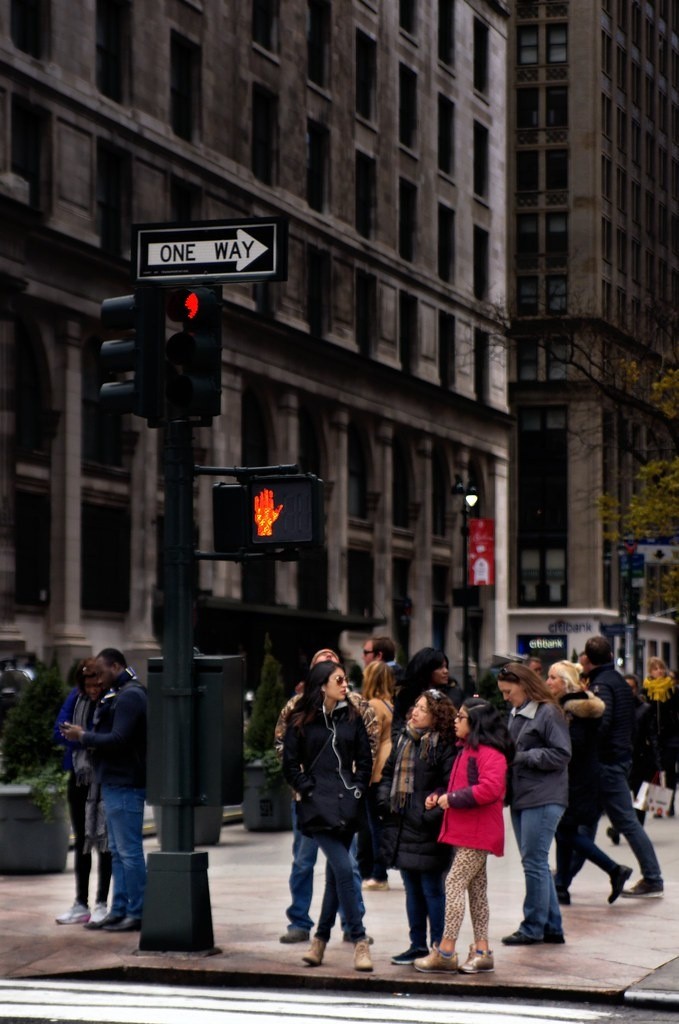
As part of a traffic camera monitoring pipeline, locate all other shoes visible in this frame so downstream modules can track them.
[344,933,374,944]
[607,827,619,845]
[279,930,309,943]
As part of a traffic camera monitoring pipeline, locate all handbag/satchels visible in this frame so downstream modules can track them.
[632,770,673,818]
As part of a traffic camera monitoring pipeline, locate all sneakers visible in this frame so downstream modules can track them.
[83,915,121,930]
[459,944,495,973]
[56,902,91,924]
[302,937,326,965]
[391,944,430,965]
[353,938,373,970]
[361,879,389,891]
[88,903,108,925]
[413,941,458,974]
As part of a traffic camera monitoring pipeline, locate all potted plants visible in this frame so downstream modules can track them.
[0,658,80,874]
[236,651,299,833]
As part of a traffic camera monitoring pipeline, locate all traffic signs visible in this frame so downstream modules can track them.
[130,215,290,283]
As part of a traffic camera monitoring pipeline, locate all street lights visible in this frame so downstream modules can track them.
[451,471,485,698]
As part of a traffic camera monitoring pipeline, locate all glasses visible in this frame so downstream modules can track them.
[328,675,348,686]
[457,713,468,723]
[363,649,373,657]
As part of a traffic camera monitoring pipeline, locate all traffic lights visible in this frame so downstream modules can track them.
[211,470,327,561]
[163,284,226,428]
[97,287,152,420]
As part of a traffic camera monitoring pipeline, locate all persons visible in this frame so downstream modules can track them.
[271,633,679,975]
[51,647,148,934]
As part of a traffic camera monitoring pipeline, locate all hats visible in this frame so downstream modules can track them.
[310,648,340,670]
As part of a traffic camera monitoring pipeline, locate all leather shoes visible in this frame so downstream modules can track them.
[620,878,664,898]
[101,917,142,932]
[502,931,542,945]
[543,934,564,943]
[556,890,570,904]
[608,865,633,903]
